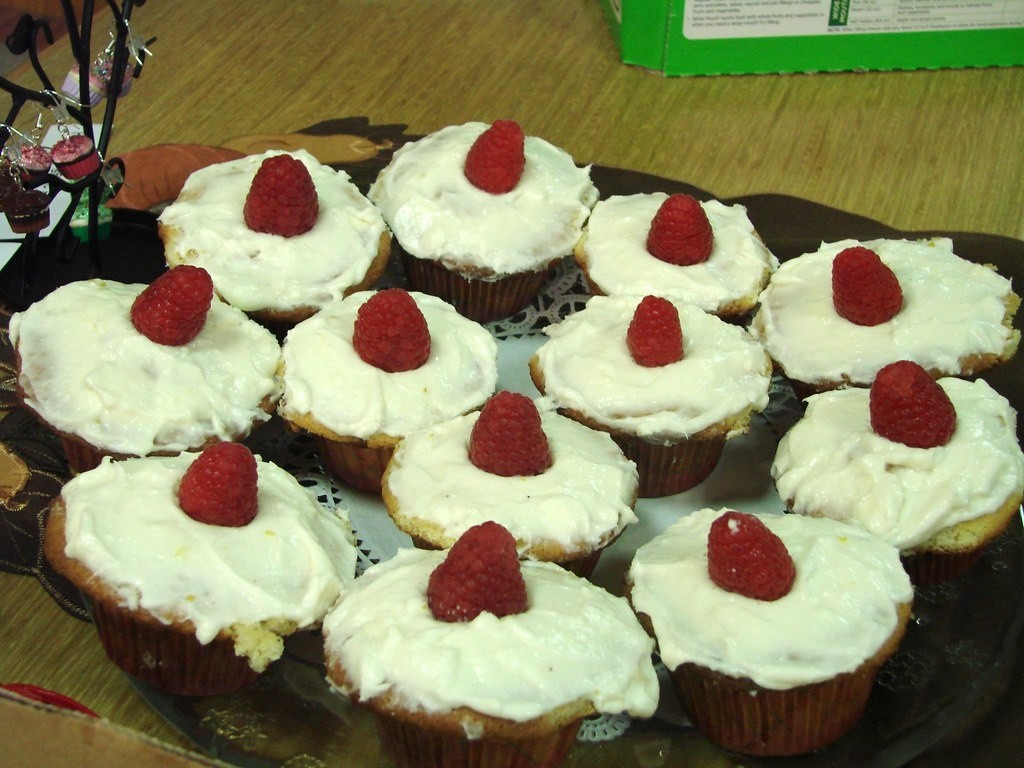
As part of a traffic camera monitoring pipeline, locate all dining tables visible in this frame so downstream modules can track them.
[0,0,1024,768]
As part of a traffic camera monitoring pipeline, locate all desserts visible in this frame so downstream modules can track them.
[4,116,1024,768]
